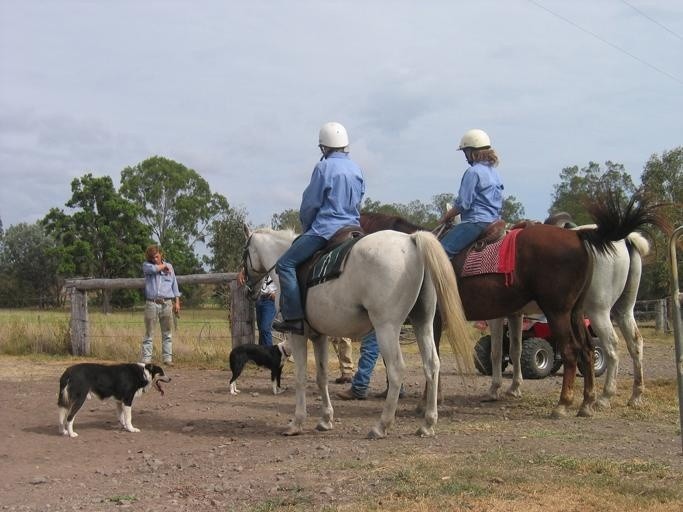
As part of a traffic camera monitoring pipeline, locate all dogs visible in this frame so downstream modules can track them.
[227,336,293,396]
[55,361,172,438]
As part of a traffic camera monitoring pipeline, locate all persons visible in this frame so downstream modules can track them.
[254,272,280,347]
[269,121,365,338]
[335,326,406,399]
[139,243,180,368]
[435,126,506,265]
[328,335,353,383]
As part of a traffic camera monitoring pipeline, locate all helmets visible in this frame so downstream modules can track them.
[457,129,491,150]
[317,121,349,149]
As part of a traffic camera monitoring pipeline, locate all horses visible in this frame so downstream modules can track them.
[361,176,680,421]
[242,223,489,441]
[479,211,652,409]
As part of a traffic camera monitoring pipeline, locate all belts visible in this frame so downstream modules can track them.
[146,296,173,304]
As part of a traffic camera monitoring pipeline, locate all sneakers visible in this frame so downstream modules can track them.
[164,361,175,367]
[375,388,407,399]
[272,319,304,336]
[334,372,367,401]
